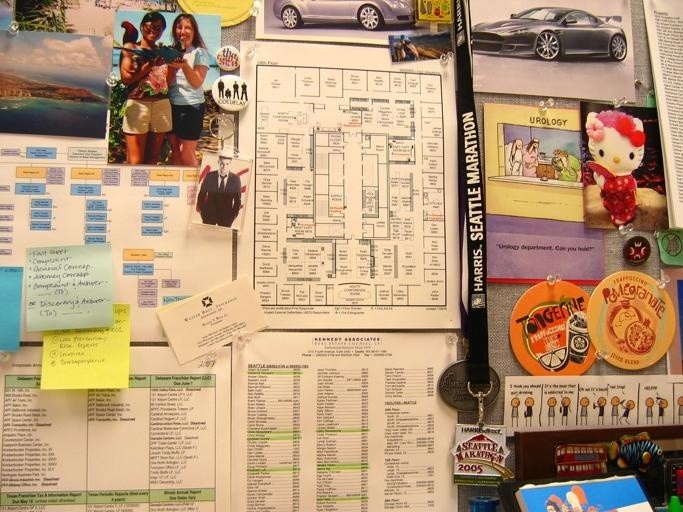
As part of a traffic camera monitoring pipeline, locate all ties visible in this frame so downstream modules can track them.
[220,174,227,192]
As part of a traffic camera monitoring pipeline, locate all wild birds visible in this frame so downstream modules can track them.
[121,21,139,46]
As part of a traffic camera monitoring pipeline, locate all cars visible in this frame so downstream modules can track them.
[469,6,627,62]
[272,1,416,30]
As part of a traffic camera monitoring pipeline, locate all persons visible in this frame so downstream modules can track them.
[118,12,184,166]
[167,13,209,167]
[217,78,247,101]
[196,155,240,228]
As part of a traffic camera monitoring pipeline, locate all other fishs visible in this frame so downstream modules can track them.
[113,42,184,62]
